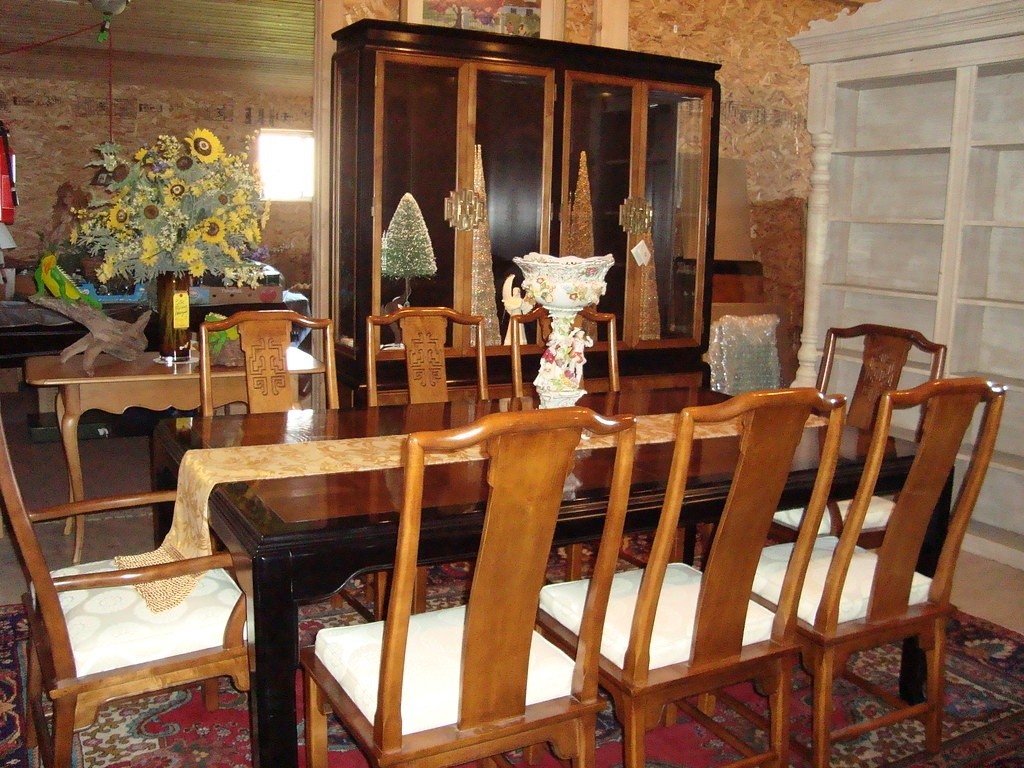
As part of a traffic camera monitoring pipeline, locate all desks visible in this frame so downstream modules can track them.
[24,344,324,562]
[150,391,955,768]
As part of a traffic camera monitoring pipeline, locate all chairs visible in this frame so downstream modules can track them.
[0,306,1007,767]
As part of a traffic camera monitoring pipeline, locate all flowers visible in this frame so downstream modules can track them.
[67,127,279,291]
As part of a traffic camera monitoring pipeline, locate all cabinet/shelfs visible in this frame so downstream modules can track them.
[332,18,723,404]
[787,0,1024,572]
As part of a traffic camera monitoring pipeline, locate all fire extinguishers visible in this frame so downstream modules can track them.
[0,121,20,224]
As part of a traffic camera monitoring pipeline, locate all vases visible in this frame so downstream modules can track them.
[149,265,201,363]
